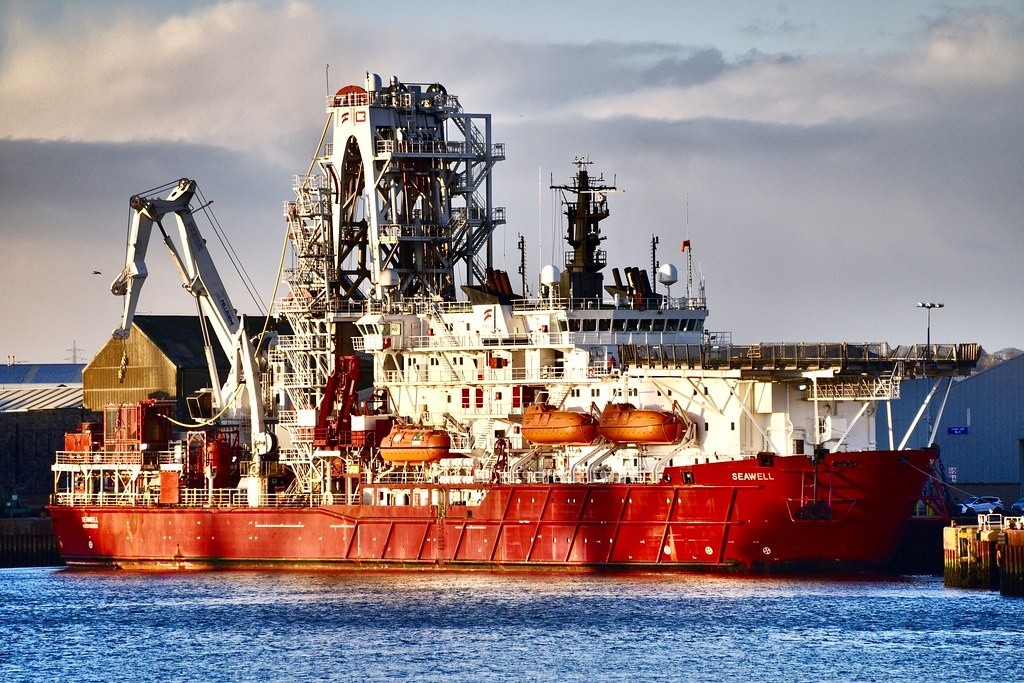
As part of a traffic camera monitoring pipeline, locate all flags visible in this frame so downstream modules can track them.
[484,309,492,321]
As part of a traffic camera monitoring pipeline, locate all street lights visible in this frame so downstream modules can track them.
[917,302,945,495]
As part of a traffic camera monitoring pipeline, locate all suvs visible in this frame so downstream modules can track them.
[1011,497,1024,514]
[955,495,1004,516]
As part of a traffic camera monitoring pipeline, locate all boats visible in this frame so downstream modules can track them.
[521,404,597,443]
[44,65,941,571]
[598,401,684,442]
[379,429,460,466]
